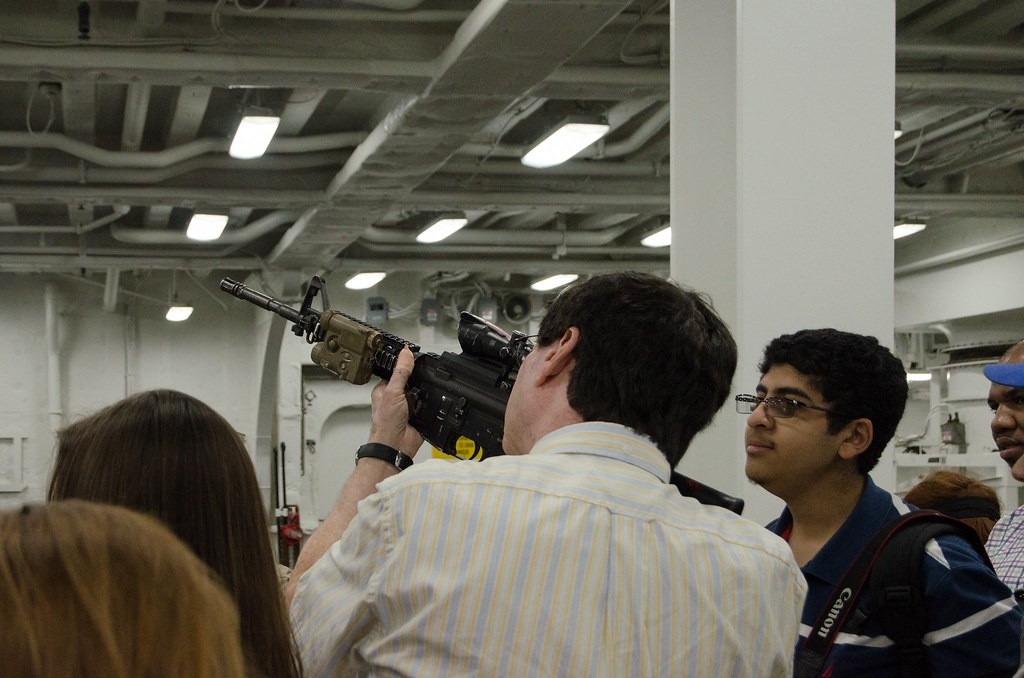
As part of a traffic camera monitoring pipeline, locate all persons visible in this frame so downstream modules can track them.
[0,388,306,677]
[743,328,1024,677]
[982,337,1024,610]
[284,270,808,678]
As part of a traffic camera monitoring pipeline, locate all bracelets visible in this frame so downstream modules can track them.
[355,443,413,471]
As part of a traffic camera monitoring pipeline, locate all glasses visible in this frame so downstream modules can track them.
[515,335,541,367]
[735,394,857,419]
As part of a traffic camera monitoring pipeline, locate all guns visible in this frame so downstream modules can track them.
[217,272,746,522]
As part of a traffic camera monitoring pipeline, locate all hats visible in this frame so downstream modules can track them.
[984,364,1024,387]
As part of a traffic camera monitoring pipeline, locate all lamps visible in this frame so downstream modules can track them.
[186,207,230,241]
[520,114,609,168]
[415,212,468,243]
[228,107,281,161]
